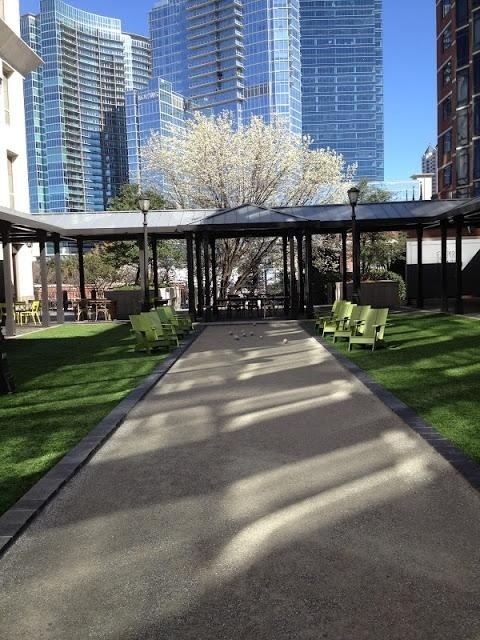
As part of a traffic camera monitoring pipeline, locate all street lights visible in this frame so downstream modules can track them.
[137,191,151,312]
[347,187,360,305]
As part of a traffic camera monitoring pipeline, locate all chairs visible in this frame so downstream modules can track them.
[314,300,389,352]
[129,305,191,356]
[0,299,43,327]
[73,295,116,323]
[200,291,279,321]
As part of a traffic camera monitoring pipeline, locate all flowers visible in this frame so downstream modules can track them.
[368,266,408,304]
